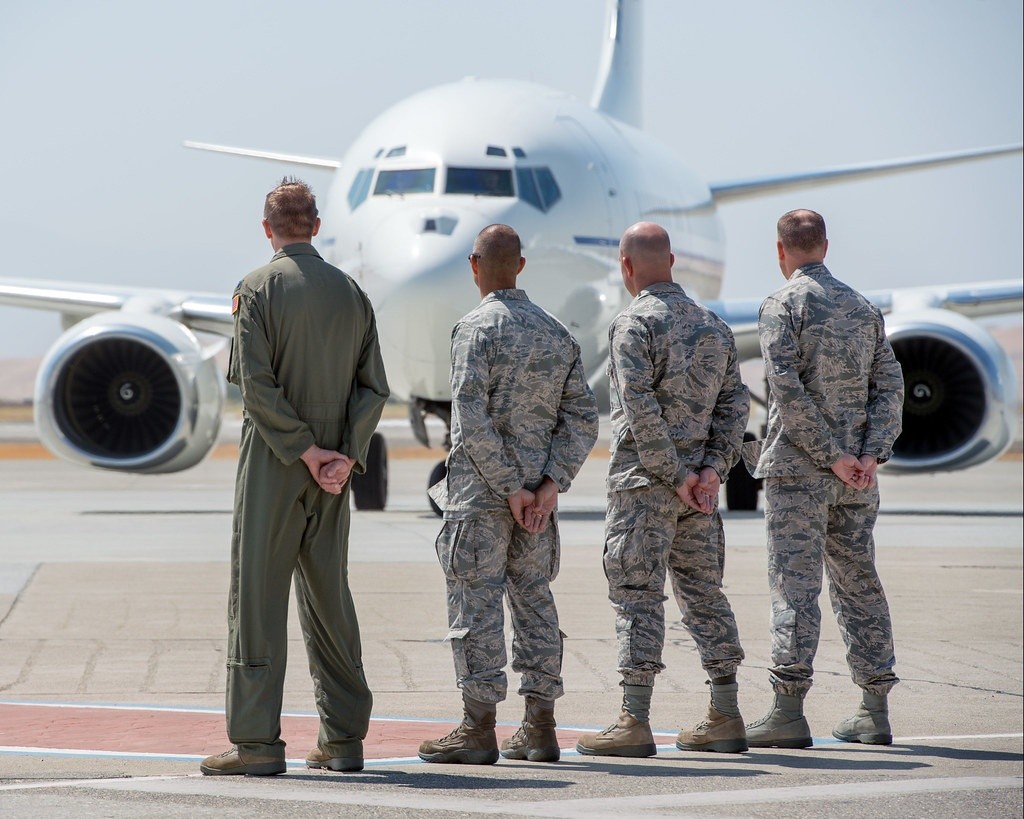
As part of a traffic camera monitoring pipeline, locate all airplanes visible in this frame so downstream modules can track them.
[0,1,1024,517]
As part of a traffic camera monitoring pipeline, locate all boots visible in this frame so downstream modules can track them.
[418,706,498,765]
[577,685,657,758]
[832,692,893,744]
[675,680,749,753]
[745,694,814,747]
[500,700,560,762]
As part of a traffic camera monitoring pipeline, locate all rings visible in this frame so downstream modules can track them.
[537,514,543,517]
[705,495,709,498]
[853,476,855,480]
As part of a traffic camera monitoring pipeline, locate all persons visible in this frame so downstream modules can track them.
[417,224,600,765]
[577,222,750,757]
[743,209,904,746]
[199,177,390,775]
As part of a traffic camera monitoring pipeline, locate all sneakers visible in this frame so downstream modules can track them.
[305,741,365,770]
[201,745,286,777]
